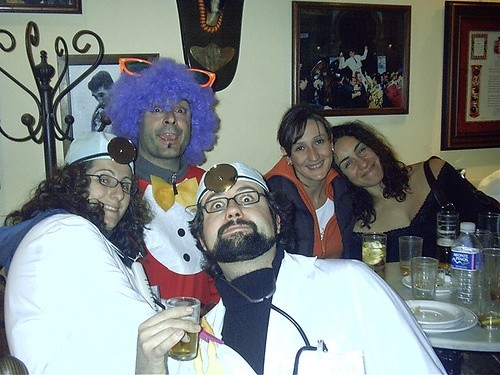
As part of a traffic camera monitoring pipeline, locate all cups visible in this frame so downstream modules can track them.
[165,296,201,361]
[399,236,424,276]
[437,209,500,331]
[362,232,387,281]
[410,257,439,301]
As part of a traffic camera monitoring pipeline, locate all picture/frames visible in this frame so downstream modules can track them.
[291,1,412,116]
[441,1,500,150]
[56,54,161,160]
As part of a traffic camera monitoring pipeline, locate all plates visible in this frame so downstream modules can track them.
[402,272,453,296]
[405,300,478,333]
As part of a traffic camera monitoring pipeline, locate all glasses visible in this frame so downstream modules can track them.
[201,190,270,214]
[86,174,138,195]
[118,57,216,88]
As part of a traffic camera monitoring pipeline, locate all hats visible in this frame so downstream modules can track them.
[196,162,270,210]
[65,130,136,175]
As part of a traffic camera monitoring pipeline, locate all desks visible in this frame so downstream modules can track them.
[384,262,500,375]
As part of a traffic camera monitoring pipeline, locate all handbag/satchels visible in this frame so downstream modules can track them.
[439,208,470,267]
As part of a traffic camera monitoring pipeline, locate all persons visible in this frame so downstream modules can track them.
[1,126,211,375]
[88,72,118,132]
[95,59,238,324]
[328,121,500,375]
[300,44,405,111]
[133,160,449,375]
[261,103,363,266]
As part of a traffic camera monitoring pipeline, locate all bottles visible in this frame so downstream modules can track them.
[449,222,485,309]
